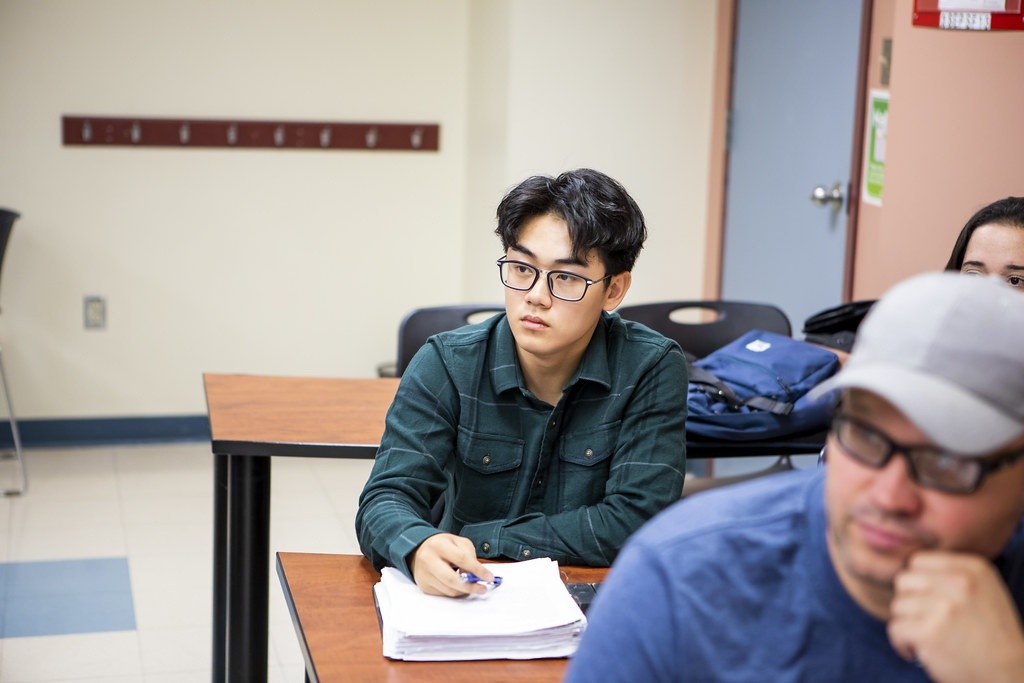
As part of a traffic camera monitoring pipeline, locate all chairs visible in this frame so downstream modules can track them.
[0,207,28,498]
[395,303,507,381]
[614,301,793,361]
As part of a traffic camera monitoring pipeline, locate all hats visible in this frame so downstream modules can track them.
[808,274,1024,455]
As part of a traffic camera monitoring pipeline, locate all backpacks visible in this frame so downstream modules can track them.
[803,301,877,334]
[685,328,844,440]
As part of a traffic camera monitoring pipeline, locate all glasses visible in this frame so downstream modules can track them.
[830,400,1024,494]
[497,255,613,302]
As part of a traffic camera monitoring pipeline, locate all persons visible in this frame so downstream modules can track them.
[944,196,1024,291]
[558,271,1024,683]
[355,169,689,598]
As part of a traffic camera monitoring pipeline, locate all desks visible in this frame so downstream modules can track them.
[274,552,613,683]
[204,377,827,682]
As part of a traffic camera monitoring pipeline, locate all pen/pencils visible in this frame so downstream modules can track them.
[458,573,502,585]
